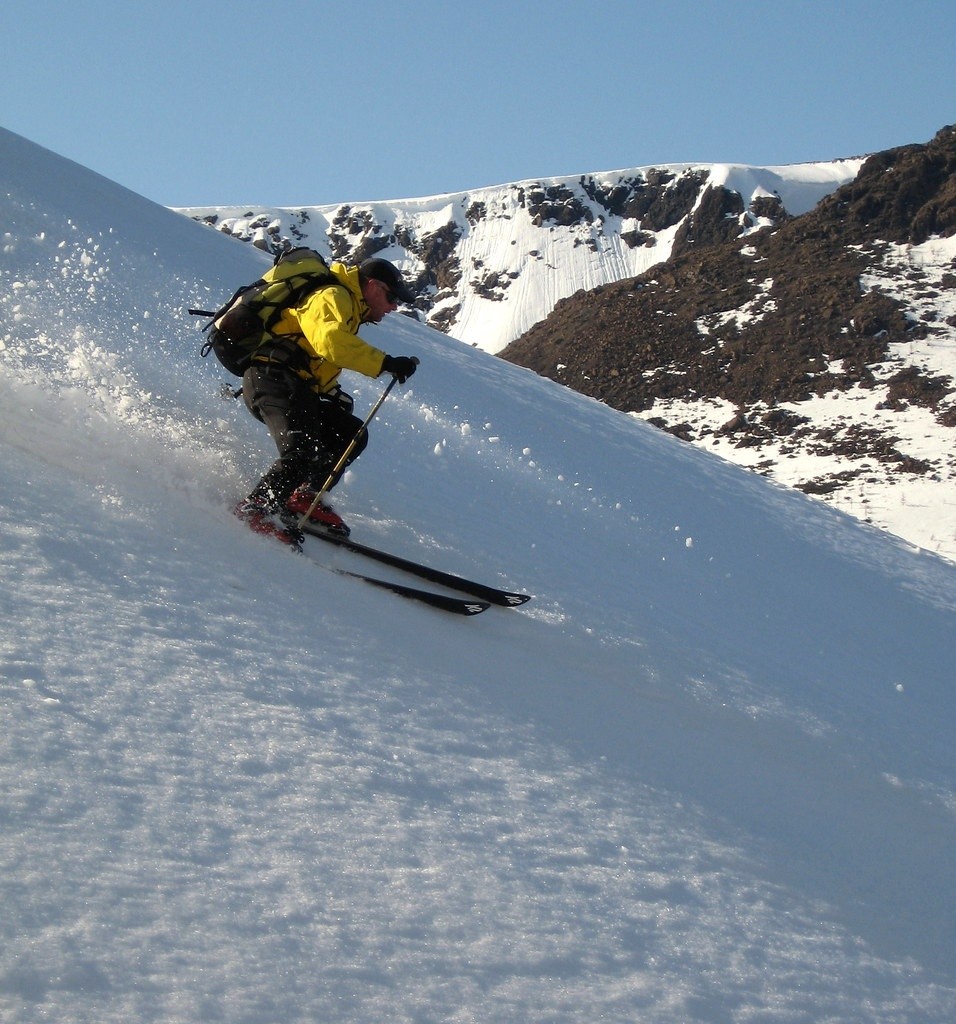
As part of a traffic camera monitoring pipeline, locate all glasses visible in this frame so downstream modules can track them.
[374,281,400,304]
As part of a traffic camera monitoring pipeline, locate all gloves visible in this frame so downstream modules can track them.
[384,354,416,384]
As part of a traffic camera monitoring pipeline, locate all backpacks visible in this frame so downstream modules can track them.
[199,247,358,377]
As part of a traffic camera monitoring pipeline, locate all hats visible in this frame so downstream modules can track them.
[360,258,416,304]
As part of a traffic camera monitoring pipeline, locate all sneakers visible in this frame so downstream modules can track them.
[287,487,350,537]
[234,497,287,543]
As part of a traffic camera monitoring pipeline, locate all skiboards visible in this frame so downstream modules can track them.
[275,515,531,616]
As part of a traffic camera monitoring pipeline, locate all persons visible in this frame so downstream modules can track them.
[233,258,420,544]
